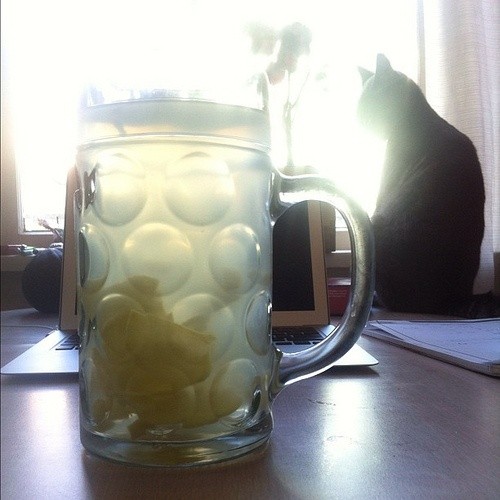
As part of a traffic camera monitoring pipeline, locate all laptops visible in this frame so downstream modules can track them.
[0,166,379,375]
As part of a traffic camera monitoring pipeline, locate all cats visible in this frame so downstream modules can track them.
[355,52,500,319]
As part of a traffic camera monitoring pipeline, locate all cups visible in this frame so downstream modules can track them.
[70,49,375,468]
